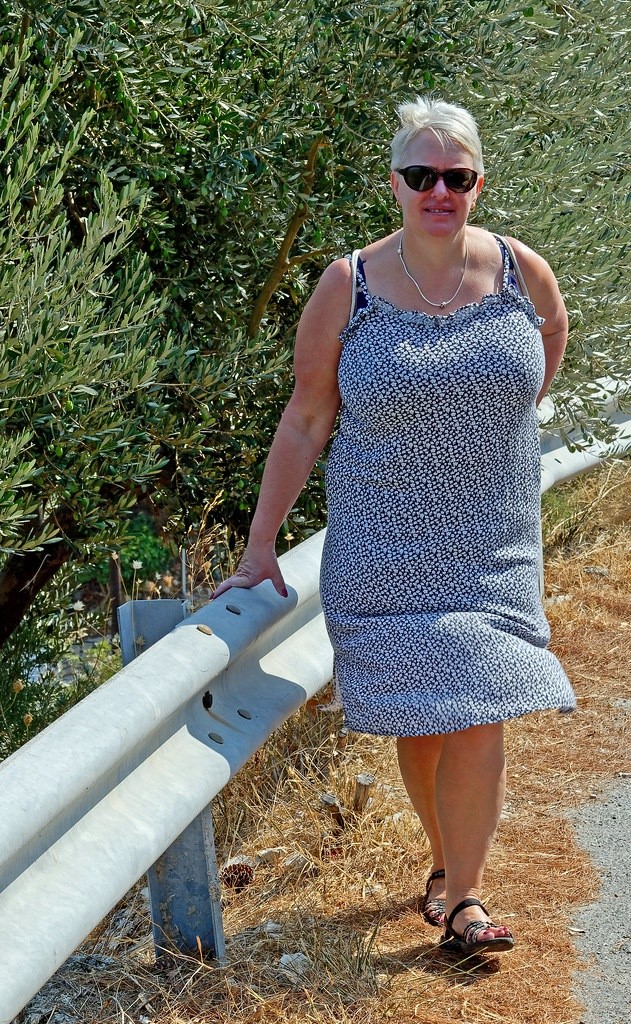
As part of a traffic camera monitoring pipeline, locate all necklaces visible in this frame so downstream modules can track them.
[397,232,468,308]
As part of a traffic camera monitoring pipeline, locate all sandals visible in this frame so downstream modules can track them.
[423,868,446,928]
[439,897,515,951]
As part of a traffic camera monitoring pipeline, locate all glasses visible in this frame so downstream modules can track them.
[395,164,477,194]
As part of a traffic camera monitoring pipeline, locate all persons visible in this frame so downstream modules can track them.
[211,97,568,956]
